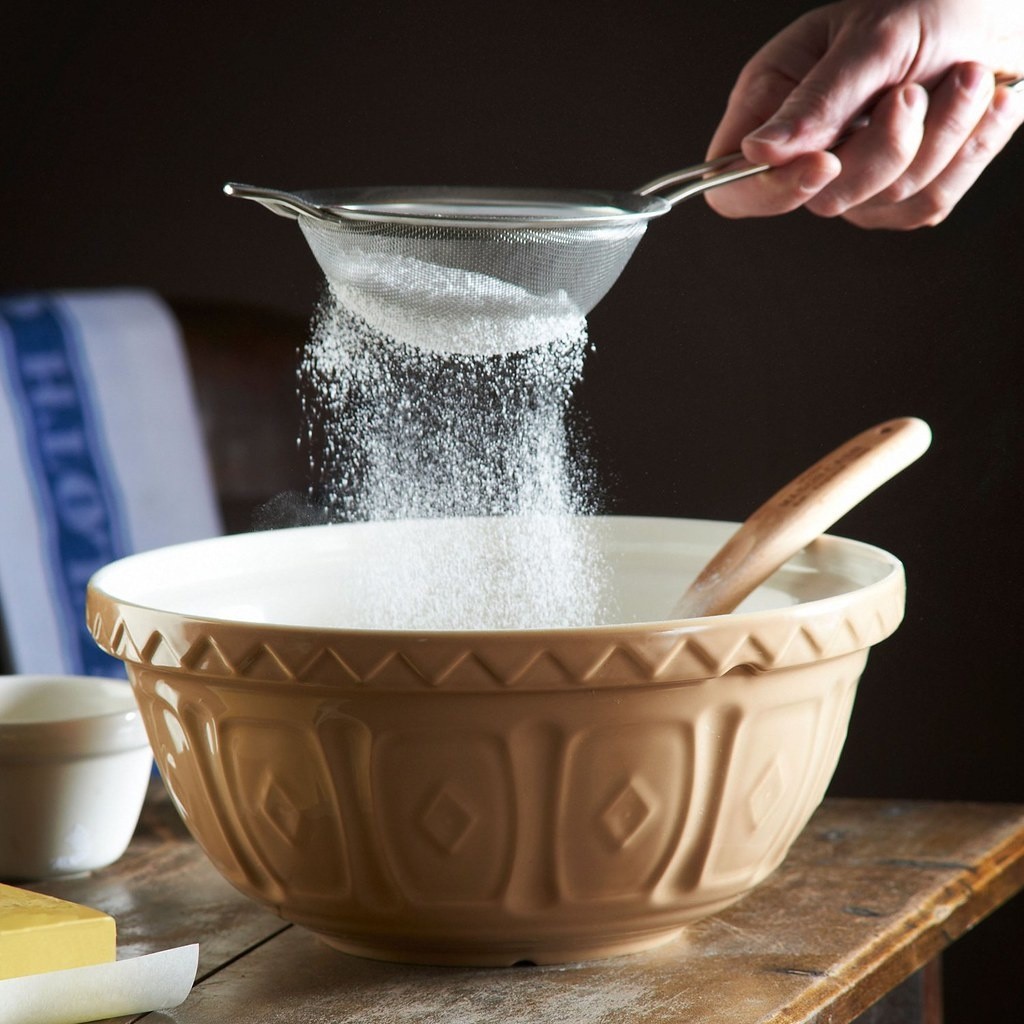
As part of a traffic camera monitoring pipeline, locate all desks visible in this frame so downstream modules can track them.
[0,796,1024,1024]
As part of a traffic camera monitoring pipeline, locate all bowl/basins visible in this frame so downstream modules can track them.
[84,515,904,967]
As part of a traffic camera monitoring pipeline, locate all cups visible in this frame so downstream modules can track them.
[0,672,155,883]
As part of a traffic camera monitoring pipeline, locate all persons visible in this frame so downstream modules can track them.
[702,0,1024,231]
